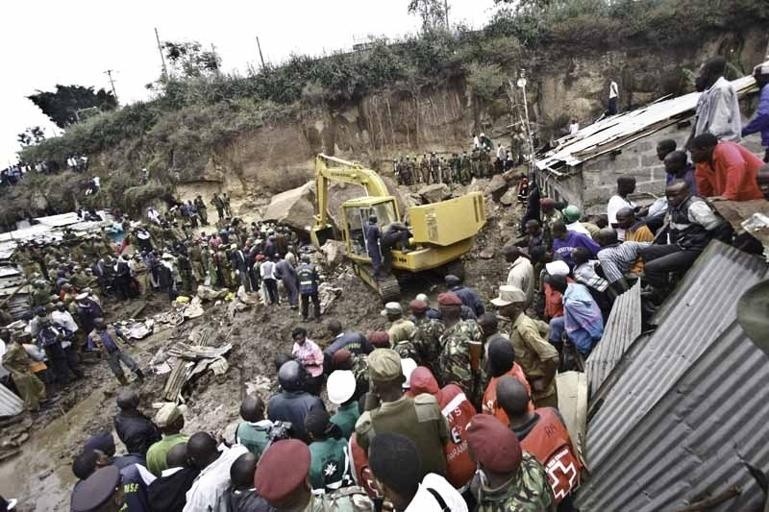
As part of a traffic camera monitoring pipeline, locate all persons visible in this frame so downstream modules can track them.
[1,55,769,512]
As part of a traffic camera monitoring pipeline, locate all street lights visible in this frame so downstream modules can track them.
[516,66,537,162]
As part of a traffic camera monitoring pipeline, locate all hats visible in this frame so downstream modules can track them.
[465,414,522,472]
[153,403,187,427]
[436,292,462,306]
[333,348,355,368]
[70,463,120,511]
[545,260,570,277]
[403,358,439,395]
[255,439,311,502]
[379,293,430,317]
[561,205,581,222]
[490,285,528,306]
[365,330,390,346]
[369,348,402,382]
[326,369,356,403]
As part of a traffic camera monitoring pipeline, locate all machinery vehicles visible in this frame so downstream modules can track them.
[303,150,489,304]
